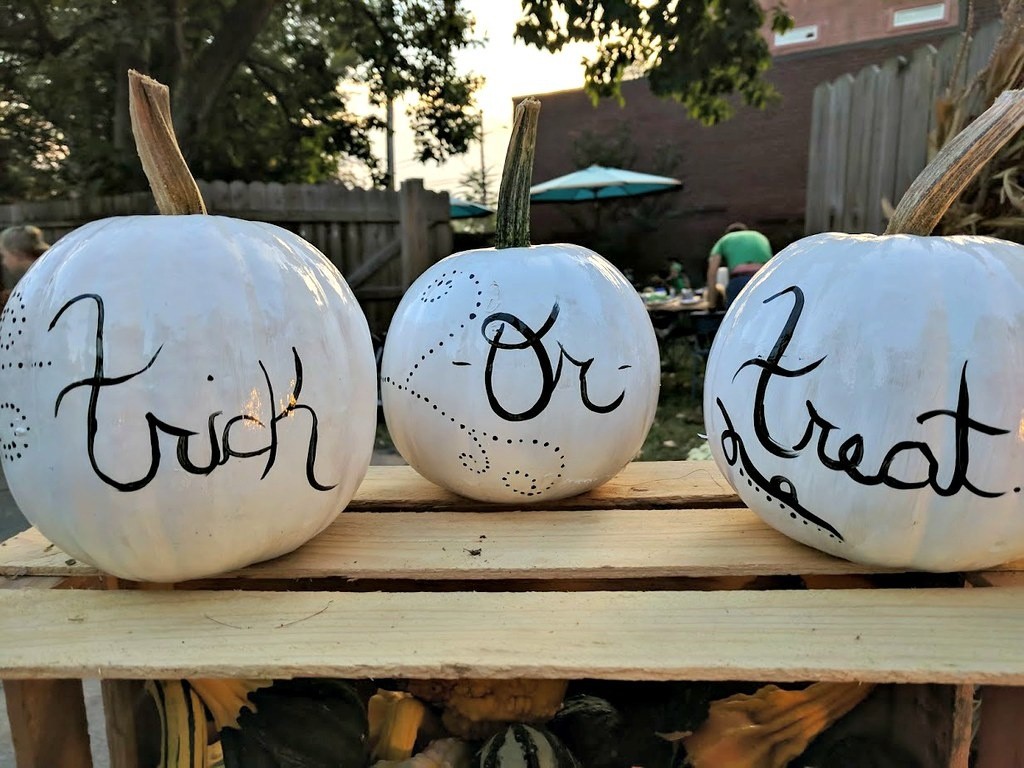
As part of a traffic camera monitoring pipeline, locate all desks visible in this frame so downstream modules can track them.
[639,291,714,372]
[0,460,1024,768]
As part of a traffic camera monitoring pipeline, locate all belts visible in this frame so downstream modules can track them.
[731,269,759,279]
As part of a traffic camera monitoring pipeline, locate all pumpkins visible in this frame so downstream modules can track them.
[0,69,382,582]
[702,91,1024,570]
[134,579,928,768]
[380,100,662,505]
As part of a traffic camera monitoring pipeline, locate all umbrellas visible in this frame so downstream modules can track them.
[450,197,495,220]
[530,165,683,258]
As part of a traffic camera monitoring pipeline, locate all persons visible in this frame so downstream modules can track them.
[706,223,773,316]
[0,225,51,312]
[647,261,691,339]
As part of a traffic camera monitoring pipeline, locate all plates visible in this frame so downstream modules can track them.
[680,296,700,305]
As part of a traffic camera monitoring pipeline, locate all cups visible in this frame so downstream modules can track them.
[682,289,693,299]
